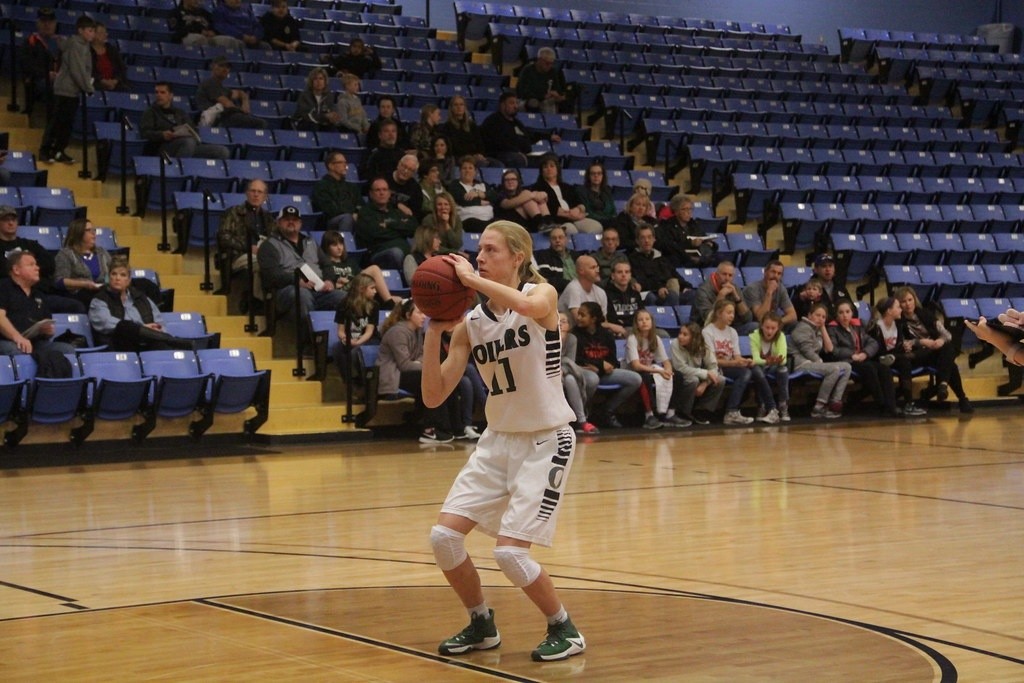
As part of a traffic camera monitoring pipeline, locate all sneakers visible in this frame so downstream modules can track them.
[531,615,586,661]
[418,430,454,444]
[438,607,501,655]
[451,432,469,440]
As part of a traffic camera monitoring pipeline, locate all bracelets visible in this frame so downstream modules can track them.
[1005,342,1024,366]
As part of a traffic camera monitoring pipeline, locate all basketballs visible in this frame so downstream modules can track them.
[410,255,476,321]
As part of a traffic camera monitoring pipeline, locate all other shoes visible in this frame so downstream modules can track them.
[464,426,482,440]
[55,151,75,163]
[576,386,974,433]
[39,153,54,163]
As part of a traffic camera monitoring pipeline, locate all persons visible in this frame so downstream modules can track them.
[88,255,220,351]
[421,220,587,660]
[10,0,971,442]
[964,307,1024,367]
[0,252,74,354]
[55,218,175,312]
[0,205,87,313]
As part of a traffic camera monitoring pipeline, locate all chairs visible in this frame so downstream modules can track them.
[0,0,1024,452]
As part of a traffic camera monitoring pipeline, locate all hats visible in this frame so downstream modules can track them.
[278,206,302,220]
[0,204,18,220]
[37,8,56,20]
[815,254,835,267]
[633,177,652,195]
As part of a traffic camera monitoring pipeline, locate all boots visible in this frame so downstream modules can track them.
[137,326,195,350]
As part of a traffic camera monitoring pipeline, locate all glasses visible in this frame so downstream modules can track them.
[85,228,96,233]
